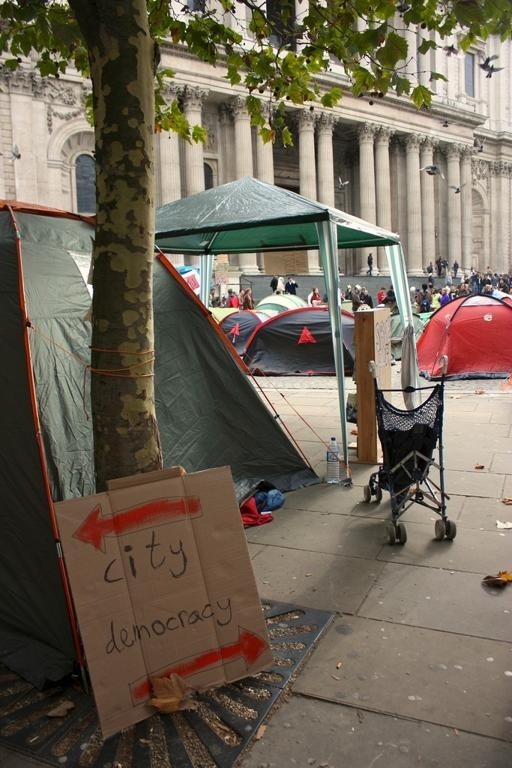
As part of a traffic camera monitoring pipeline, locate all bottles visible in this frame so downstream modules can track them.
[326,436,340,483]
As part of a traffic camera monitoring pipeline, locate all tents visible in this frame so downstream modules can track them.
[154,177,421,485]
[216,291,509,381]
[2,197,324,690]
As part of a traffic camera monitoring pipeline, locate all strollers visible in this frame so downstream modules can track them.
[363,383,461,544]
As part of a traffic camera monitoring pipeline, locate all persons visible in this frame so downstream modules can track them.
[378,256,512,312]
[308,285,373,311]
[208,286,254,310]
[270,275,298,295]
[366,253,373,276]
